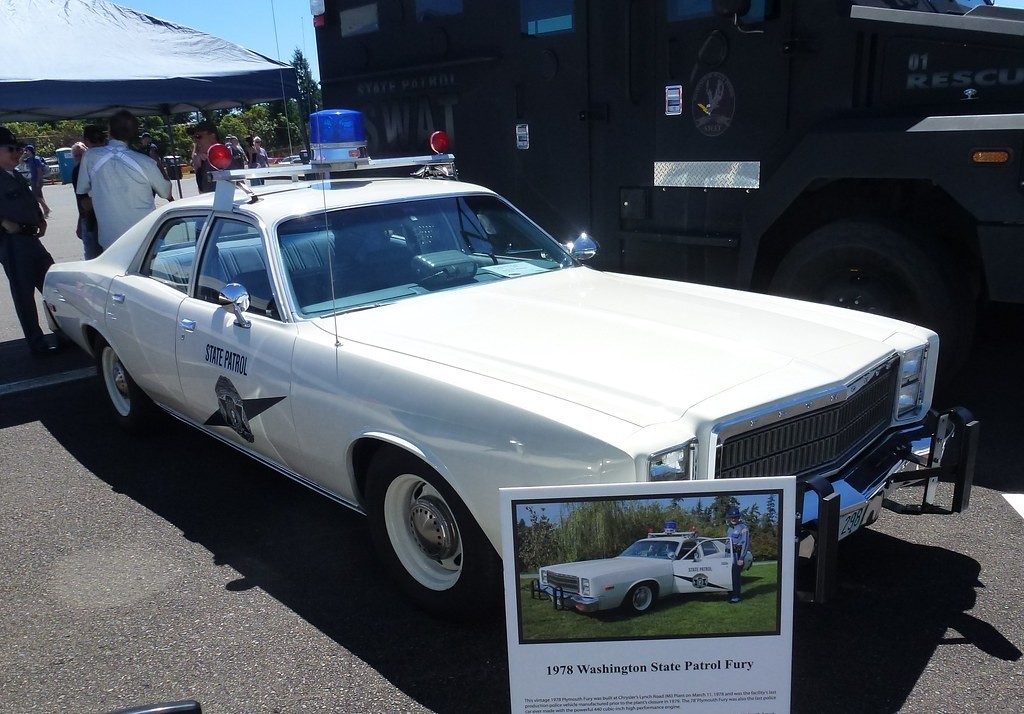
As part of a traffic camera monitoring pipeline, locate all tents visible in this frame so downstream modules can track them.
[0,0,310,158]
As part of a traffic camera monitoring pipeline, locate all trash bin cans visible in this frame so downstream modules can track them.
[164,155,183,180]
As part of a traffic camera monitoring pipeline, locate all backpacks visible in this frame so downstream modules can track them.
[33,156,49,174]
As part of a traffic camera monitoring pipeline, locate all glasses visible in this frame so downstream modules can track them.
[191,132,212,141]
[0,146,21,152]
[88,137,104,143]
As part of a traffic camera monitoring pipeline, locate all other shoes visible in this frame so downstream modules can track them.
[44,210,50,218]
[58,340,76,348]
[32,346,58,356]
[731,598,741,602]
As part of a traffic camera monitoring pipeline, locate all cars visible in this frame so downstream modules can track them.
[14,157,60,182]
[43,0,980,619]
[531,523,752,623]
[266,154,304,165]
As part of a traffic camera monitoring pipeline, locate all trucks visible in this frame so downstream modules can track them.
[309,0,1024,413]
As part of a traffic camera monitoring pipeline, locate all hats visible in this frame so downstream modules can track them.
[139,132,153,139]
[726,508,741,518]
[186,121,218,135]
[23,145,35,152]
[226,134,232,138]
[83,122,105,137]
[0,127,22,147]
[244,137,253,144]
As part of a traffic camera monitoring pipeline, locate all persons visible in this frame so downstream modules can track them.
[0,124,55,346]
[724,508,753,602]
[190,120,269,240]
[70,110,171,260]
